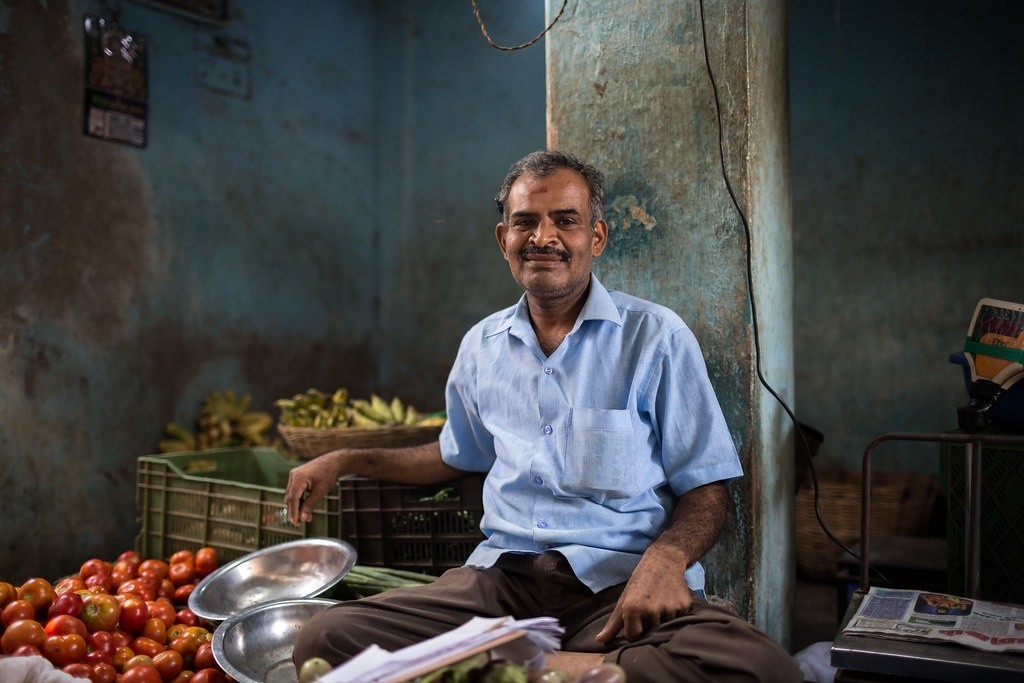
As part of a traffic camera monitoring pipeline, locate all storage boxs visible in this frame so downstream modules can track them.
[278,425,444,456]
[792,471,949,575]
[136,445,487,568]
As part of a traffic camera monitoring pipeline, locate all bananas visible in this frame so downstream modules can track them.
[159,385,447,472]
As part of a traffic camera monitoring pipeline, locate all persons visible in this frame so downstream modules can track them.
[286,150,800,683]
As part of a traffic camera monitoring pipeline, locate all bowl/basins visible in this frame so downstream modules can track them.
[188,535,358,625]
[211,598,344,683]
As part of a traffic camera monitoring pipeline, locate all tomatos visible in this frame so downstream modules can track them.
[1,548,234,683]
